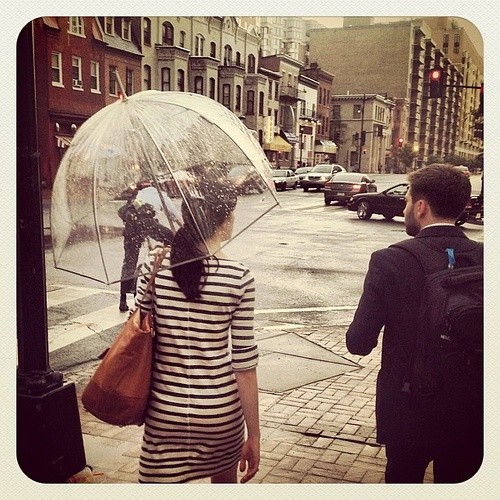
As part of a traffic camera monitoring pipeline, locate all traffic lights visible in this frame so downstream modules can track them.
[377,125,382,136]
[399,137,403,147]
[478,83,484,117]
[430,68,442,98]
[361,130,366,146]
[473,123,484,141]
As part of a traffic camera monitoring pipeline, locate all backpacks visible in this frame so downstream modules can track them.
[389,240,483,408]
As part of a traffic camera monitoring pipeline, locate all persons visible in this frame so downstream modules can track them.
[129,181,261,484]
[346,164,484,484]
[118,185,184,313]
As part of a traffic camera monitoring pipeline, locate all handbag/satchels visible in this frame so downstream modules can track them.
[80,247,170,427]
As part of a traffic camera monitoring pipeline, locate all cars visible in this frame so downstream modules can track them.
[324,172,377,206]
[267,170,300,191]
[295,167,314,186]
[300,164,347,192]
[347,183,410,221]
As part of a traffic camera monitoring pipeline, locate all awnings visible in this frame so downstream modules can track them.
[55,135,114,148]
[284,132,299,144]
[265,136,293,153]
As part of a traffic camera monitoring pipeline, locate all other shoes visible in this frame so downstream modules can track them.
[119,304,129,311]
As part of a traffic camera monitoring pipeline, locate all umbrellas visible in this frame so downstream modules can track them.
[50,66,282,285]
